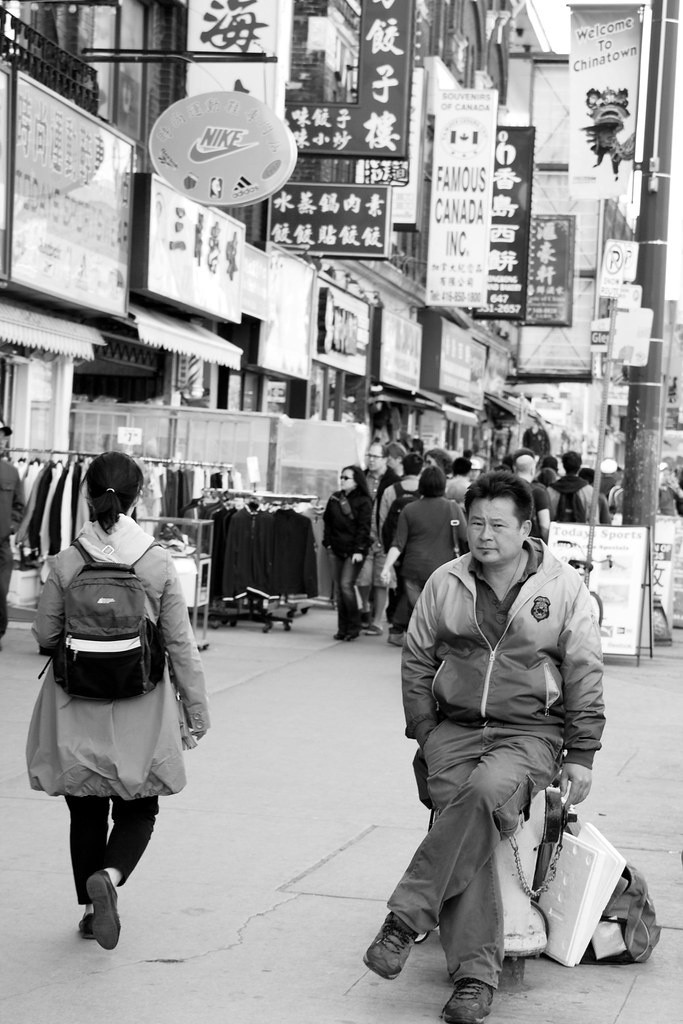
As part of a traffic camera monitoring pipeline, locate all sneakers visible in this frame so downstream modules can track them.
[439,978,494,1024]
[362,911,418,980]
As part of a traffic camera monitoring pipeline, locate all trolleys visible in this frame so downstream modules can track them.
[138,514,215,652]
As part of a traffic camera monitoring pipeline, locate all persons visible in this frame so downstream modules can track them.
[0,419,27,649]
[521,422,550,463]
[364,470,605,1024]
[577,466,611,524]
[362,439,403,635]
[473,420,492,454]
[377,453,422,646]
[489,423,513,466]
[25,451,210,950]
[511,455,551,545]
[378,439,683,556]
[546,451,601,525]
[379,466,471,607]
[322,465,373,643]
[590,458,621,501]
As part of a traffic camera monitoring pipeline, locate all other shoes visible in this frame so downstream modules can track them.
[387,632,404,646]
[344,634,360,640]
[366,625,383,635]
[333,633,345,640]
[87,869,121,949]
[77,913,95,939]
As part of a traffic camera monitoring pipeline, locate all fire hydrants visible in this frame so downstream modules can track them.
[496,783,579,989]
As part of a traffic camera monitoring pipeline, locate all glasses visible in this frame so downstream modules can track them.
[366,453,384,459]
[340,476,354,481]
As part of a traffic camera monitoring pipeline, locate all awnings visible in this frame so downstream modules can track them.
[127,300,244,371]
[487,392,553,427]
[0,297,107,363]
[385,380,479,430]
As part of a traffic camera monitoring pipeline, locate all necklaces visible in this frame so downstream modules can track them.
[499,552,521,603]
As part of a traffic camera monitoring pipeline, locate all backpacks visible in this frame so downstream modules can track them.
[553,489,587,524]
[583,863,661,963]
[52,538,164,702]
[381,481,423,553]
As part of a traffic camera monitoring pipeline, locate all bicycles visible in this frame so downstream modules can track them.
[554,537,612,629]
